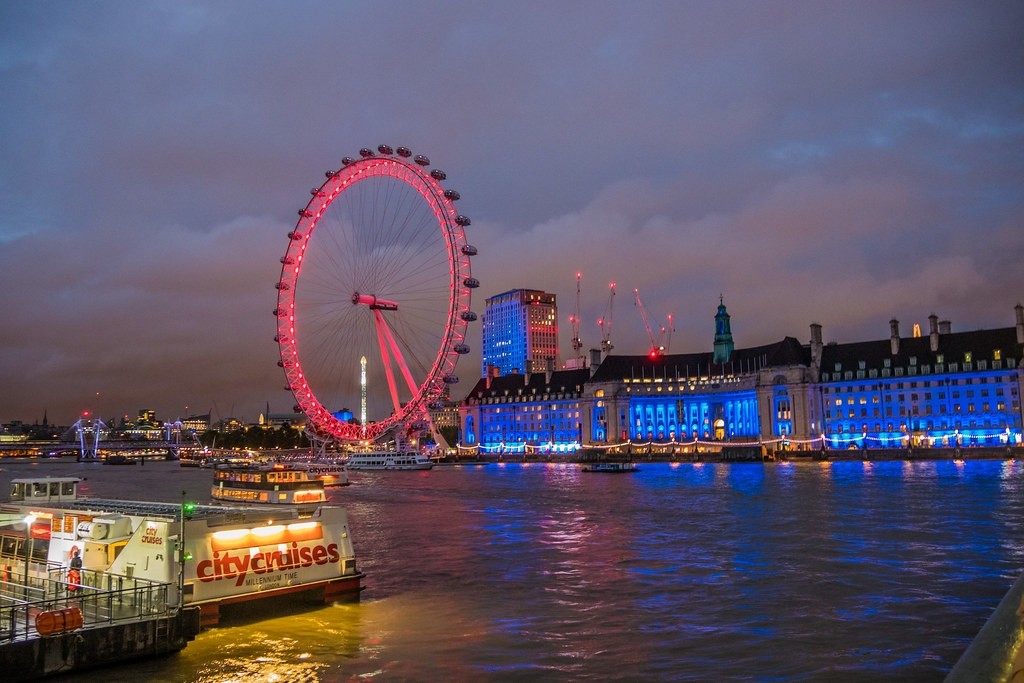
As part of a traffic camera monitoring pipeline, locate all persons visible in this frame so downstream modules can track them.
[70,551,82,571]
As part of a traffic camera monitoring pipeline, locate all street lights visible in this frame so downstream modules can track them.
[23,516,36,609]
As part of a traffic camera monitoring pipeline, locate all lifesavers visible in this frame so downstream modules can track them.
[66,571,81,592]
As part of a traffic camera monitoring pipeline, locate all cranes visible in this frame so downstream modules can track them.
[632,288,665,357]
[666,315,674,353]
[569,273,583,368]
[599,282,616,356]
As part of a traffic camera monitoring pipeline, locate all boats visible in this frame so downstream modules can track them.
[0,475,366,628]
[211,466,329,515]
[255,463,349,487]
[582,462,640,473]
[344,450,434,470]
